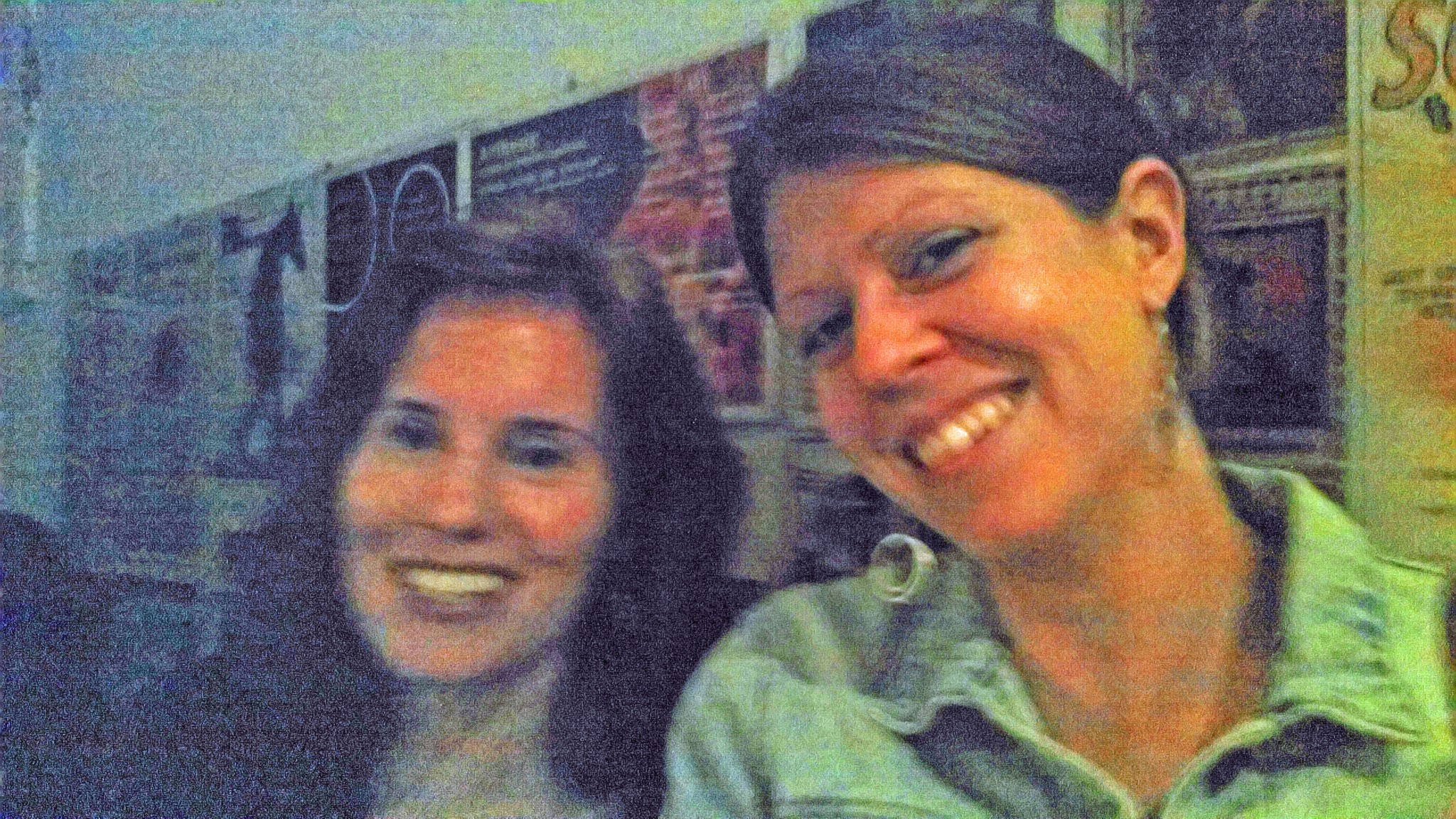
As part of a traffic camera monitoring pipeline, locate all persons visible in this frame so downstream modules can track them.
[657,10,1456,819]
[103,191,752,819]
